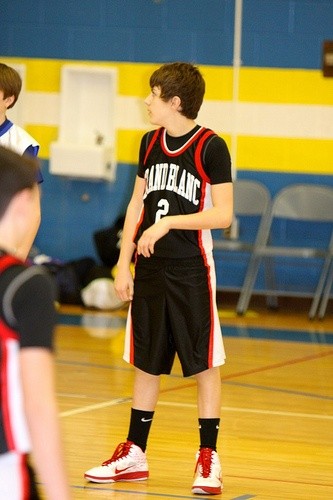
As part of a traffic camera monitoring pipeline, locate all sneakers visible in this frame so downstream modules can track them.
[83,441,148,483]
[191,448,222,494]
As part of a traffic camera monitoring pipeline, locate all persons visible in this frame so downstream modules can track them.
[83,63,234,493]
[0,145,72,500]
[0,62,42,184]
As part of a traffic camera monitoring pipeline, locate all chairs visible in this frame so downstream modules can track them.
[238,185,333,320]
[208,181,271,315]
